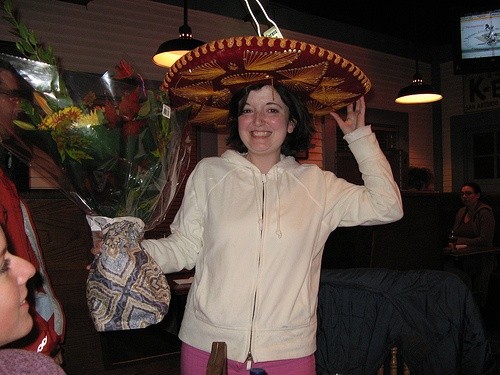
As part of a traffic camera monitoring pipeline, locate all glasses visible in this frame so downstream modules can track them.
[0,89,23,97]
[460,191,474,194]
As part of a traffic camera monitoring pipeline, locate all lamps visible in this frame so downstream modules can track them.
[152,1,210,71]
[394,55,443,105]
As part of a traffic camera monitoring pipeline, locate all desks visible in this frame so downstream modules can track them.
[441,245,499,269]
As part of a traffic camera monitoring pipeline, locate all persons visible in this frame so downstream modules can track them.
[446,182,495,313]
[87,78,403,375]
[0,60,68,375]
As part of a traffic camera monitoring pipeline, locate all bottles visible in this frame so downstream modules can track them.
[448,230,457,254]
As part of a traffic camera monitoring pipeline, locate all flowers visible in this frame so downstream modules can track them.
[0,1,196,220]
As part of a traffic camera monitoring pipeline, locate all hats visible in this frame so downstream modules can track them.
[161,34,372,132]
